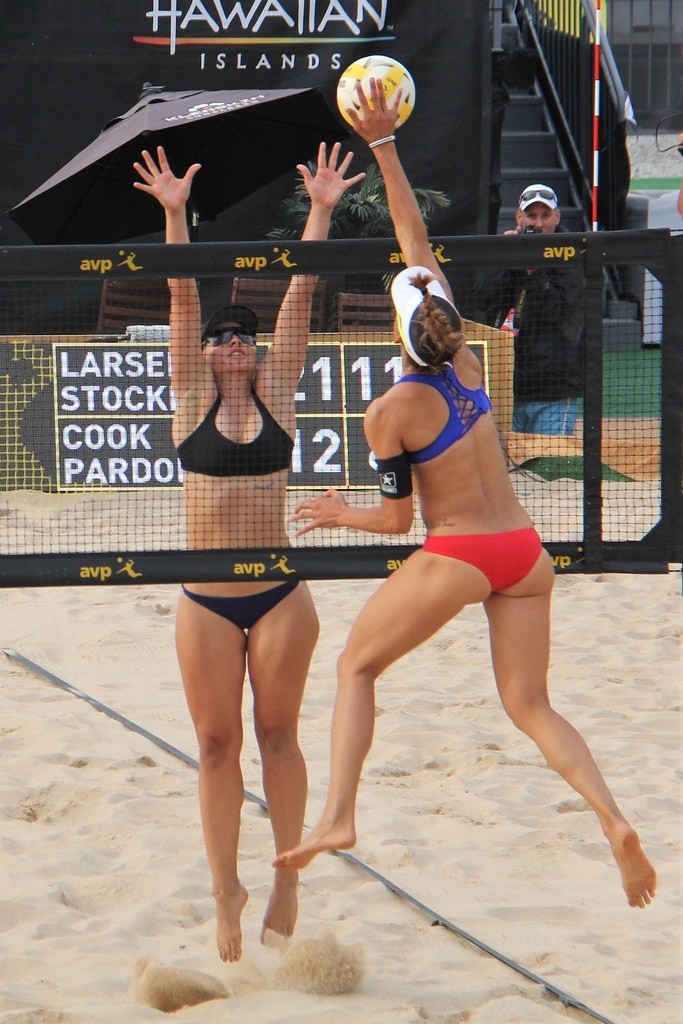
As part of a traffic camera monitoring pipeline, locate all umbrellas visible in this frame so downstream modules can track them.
[4,82,352,244]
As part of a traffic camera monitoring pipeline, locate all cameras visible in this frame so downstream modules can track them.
[517,225,542,234]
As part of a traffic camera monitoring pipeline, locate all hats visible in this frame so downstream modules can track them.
[390,265,464,368]
[200,305,259,345]
[518,183,558,211]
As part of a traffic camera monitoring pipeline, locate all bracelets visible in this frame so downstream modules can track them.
[370,135,396,147]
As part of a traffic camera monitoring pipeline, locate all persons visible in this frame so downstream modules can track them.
[472,183,586,434]
[270,78,662,909]
[676,133,683,215]
[133,142,367,962]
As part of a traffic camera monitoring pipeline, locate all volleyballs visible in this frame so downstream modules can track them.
[336,55,415,131]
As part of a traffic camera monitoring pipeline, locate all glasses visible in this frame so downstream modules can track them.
[519,190,557,209]
[202,327,256,347]
